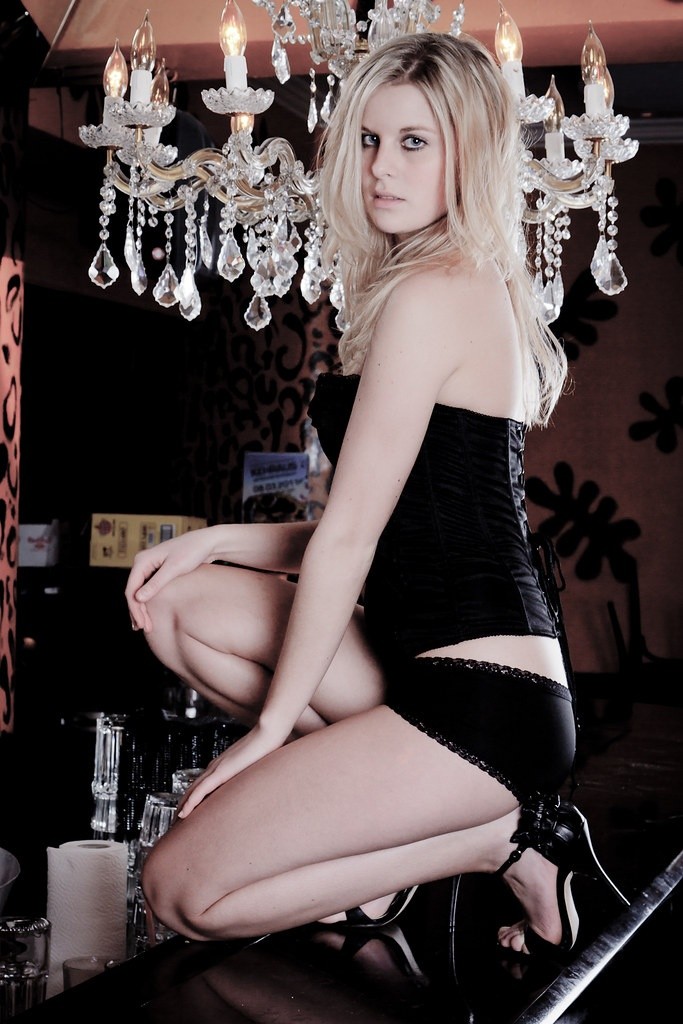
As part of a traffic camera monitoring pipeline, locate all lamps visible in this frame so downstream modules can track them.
[78,1,639,334]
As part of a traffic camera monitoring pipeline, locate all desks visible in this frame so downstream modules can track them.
[0,701,683,1024]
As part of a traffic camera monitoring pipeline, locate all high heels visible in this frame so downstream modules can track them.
[491,803,634,969]
[329,932,424,980]
[319,881,411,929]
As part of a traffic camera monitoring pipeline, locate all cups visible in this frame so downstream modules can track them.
[0,916,51,1020]
[63,955,128,990]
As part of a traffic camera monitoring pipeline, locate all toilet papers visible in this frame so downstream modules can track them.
[43,839,128,1000]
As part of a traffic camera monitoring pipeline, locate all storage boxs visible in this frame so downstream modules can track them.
[88,514,207,567]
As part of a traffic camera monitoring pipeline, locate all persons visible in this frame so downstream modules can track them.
[179,927,474,1024]
[124,31,630,957]
